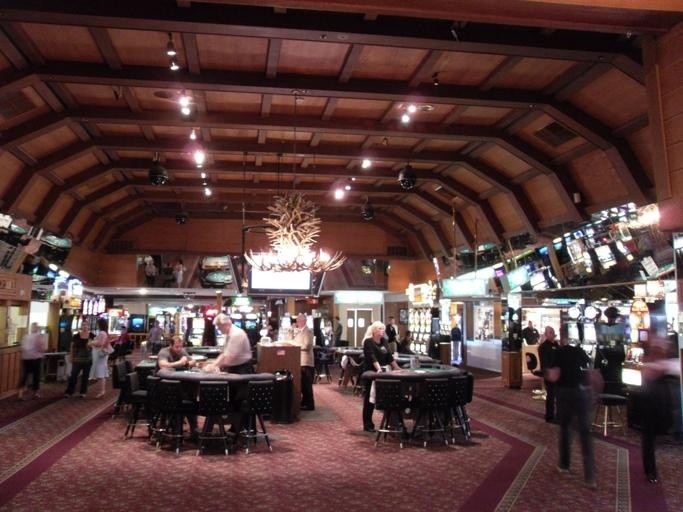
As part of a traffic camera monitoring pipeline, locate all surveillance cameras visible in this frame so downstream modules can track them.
[396,168,418,190]
[359,203,377,220]
[148,163,169,186]
[175,209,188,224]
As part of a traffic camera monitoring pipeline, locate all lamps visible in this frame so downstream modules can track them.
[359,196,374,220]
[164,29,215,199]
[243,88,348,273]
[567,305,602,323]
[176,211,188,225]
[428,71,438,85]
[144,150,169,185]
[395,157,417,190]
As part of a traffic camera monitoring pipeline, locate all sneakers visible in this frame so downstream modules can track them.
[648,477,657,483]
[584,481,597,489]
[555,463,570,474]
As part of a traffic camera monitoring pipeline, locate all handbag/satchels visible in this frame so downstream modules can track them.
[98,333,114,357]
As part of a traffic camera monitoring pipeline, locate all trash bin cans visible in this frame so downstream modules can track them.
[270,367,294,424]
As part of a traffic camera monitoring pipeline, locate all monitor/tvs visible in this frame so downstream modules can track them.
[227,255,244,294]
[244,319,260,332]
[232,320,243,330]
[248,252,312,295]
[313,272,326,296]
[130,314,146,332]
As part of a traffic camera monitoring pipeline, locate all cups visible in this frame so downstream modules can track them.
[184,361,221,375]
[410,356,420,370]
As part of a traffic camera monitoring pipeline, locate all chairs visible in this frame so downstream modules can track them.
[577,364,630,439]
[315,339,364,397]
[110,336,280,456]
[363,353,474,449]
[524,351,550,401]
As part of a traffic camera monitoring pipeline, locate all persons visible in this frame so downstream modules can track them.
[451,323,461,360]
[146,260,185,288]
[203,313,256,432]
[18,323,45,401]
[63,317,129,398]
[157,335,196,430]
[329,316,343,346]
[283,312,314,410]
[148,320,164,356]
[359,315,416,433]
[520,311,683,487]
[287,316,298,340]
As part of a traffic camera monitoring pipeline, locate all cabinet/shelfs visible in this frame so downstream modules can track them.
[405,307,439,360]
